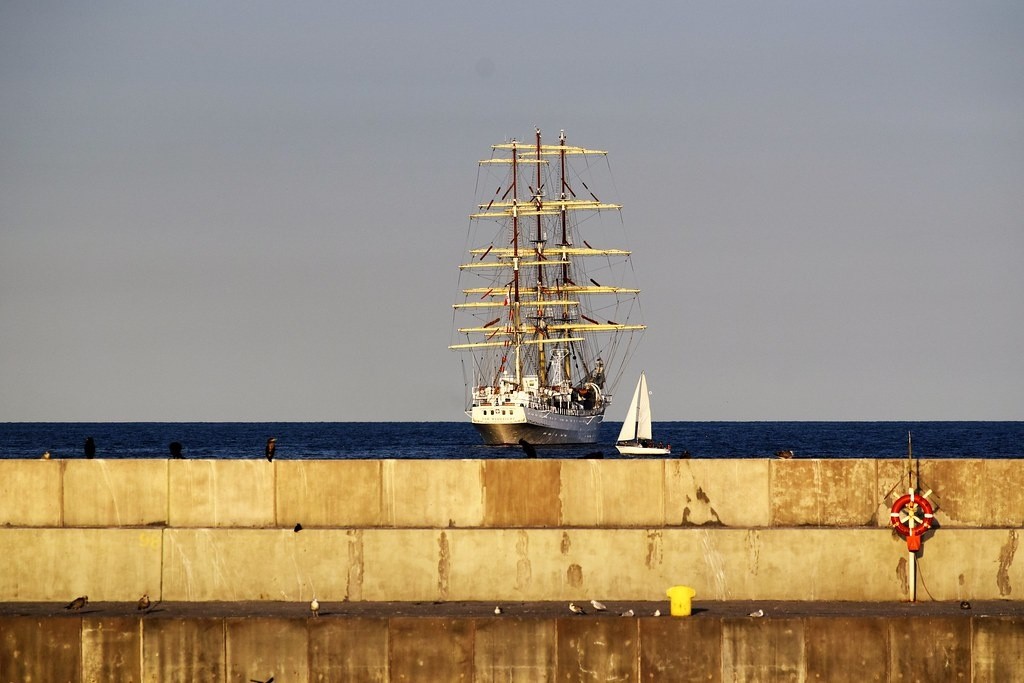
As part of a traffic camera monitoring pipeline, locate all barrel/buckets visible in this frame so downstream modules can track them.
[667,586,696,616]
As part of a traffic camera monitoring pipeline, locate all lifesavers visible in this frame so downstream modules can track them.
[891,493,935,537]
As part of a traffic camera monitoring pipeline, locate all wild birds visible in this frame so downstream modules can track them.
[569,602,585,615]
[166,441,186,459]
[960,600,971,610]
[310,599,320,617]
[62,595,88,610]
[590,600,607,613]
[772,449,794,459]
[265,437,278,462]
[138,593,150,613]
[747,609,764,618]
[493,605,500,615]
[84,436,96,460]
[619,609,660,617]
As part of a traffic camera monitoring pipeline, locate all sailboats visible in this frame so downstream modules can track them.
[614,370,672,455]
[448,126,648,445]
